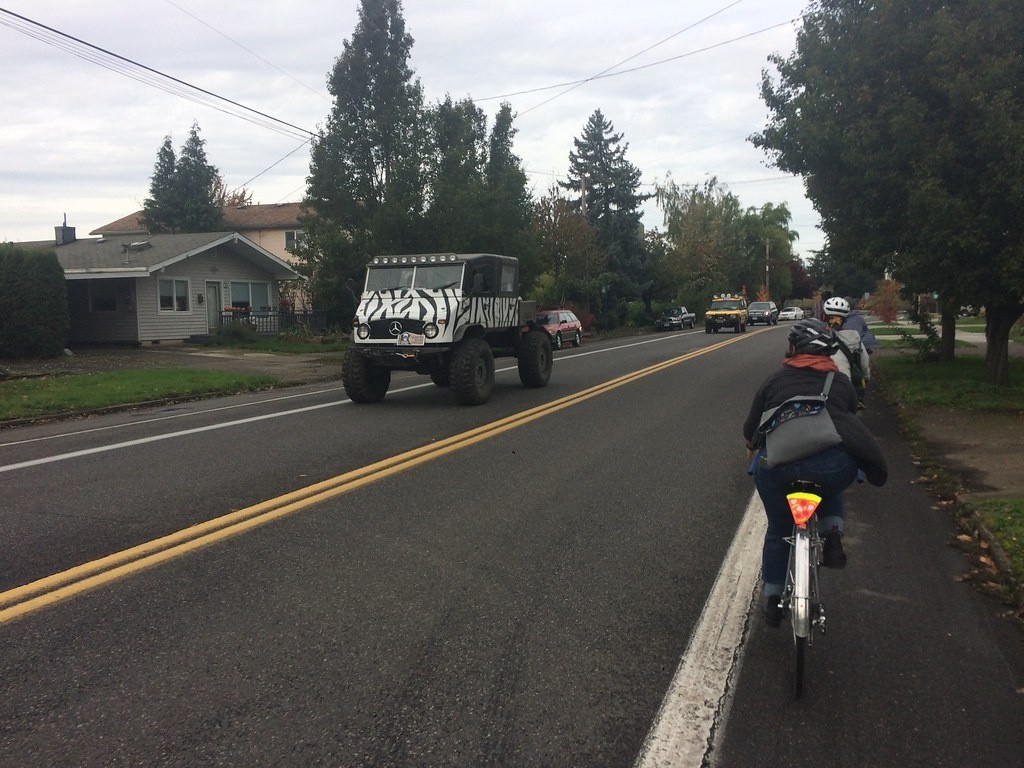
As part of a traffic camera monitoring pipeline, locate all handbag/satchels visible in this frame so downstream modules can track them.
[850,349,865,387]
[758,371,842,468]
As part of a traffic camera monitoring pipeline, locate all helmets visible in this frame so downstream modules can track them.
[787,317,840,355]
[823,297,850,317]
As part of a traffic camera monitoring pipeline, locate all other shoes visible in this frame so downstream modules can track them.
[761,597,783,627]
[823,525,846,569]
[857,399,866,409]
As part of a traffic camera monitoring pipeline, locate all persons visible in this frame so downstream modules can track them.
[840,296,876,357]
[814,290,833,321]
[742,317,864,631]
[819,296,872,389]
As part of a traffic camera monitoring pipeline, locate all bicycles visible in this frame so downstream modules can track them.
[777,479,826,698]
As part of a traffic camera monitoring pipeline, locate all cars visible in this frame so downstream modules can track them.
[777,307,804,320]
[961,305,978,317]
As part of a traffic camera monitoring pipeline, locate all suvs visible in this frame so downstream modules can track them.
[705,294,749,333]
[522,309,582,350]
[342,251,552,407]
[746,301,779,325]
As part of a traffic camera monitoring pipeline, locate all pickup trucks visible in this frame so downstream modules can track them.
[655,307,696,330]
